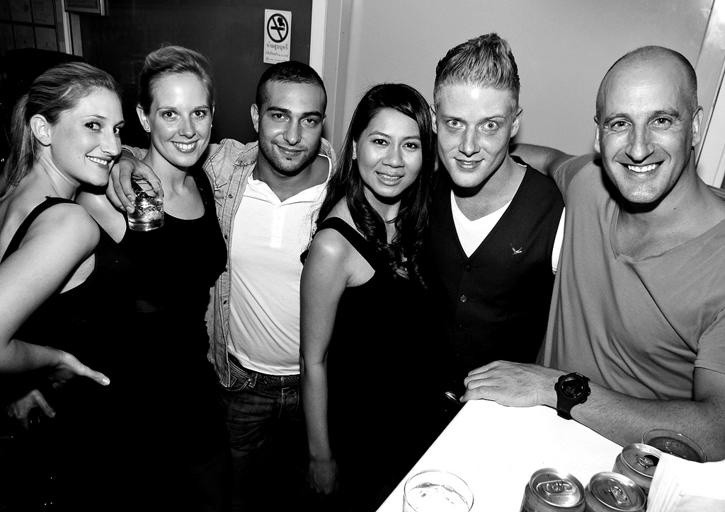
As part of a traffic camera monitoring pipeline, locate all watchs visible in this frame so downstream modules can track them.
[554,371,591,420]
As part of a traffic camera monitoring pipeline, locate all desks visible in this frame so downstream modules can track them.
[375,400,622,512]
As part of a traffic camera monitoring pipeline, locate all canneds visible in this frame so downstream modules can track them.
[615,443,662,493]
[587,471,647,510]
[521,467,586,512]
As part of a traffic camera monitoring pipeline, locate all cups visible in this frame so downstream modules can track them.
[128,179,164,232]
[403,470,475,512]
[643,429,706,462]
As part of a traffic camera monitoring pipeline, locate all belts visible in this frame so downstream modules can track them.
[231,374,301,407]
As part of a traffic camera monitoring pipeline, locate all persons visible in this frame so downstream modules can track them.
[411,34,566,408]
[0,50,128,510]
[298,82,444,495]
[458,45,724,464]
[103,60,346,511]
[9,44,229,512]
